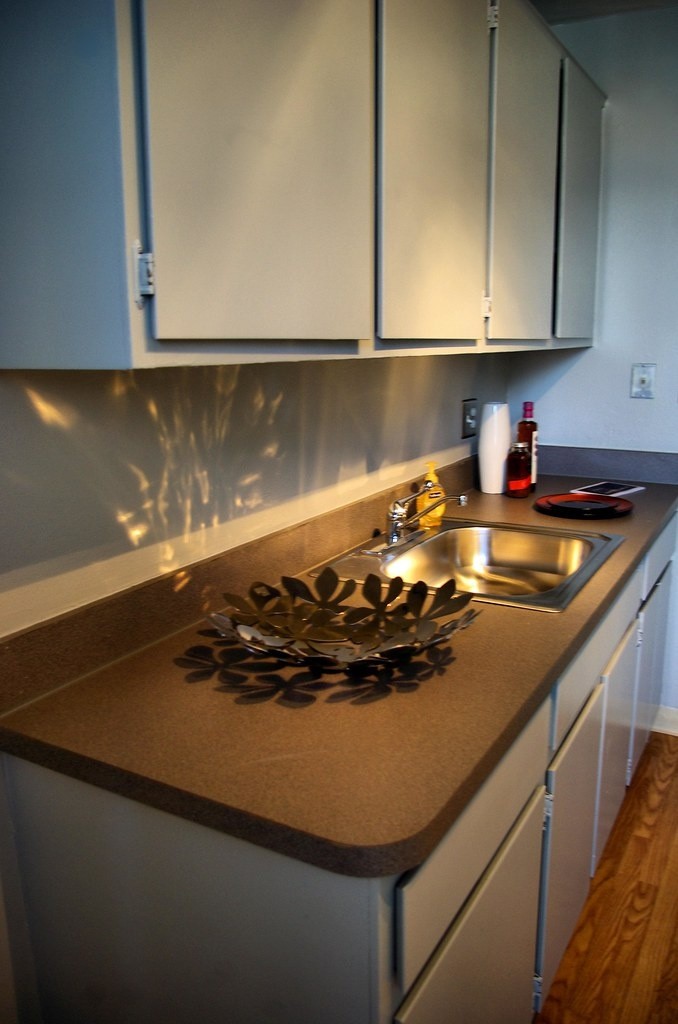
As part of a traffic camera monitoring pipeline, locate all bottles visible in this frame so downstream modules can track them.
[507,442,531,498]
[517,402,538,494]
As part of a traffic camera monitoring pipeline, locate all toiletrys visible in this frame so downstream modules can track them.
[415,461,446,527]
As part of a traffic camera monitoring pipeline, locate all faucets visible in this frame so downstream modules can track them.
[387,479,468,554]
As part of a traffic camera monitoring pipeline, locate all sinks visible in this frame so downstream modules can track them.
[305,519,627,613]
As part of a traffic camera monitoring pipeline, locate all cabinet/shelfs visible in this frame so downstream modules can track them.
[0,0,375,372]
[553,48,608,350]
[0,511,678,1024]
[373,2,563,355]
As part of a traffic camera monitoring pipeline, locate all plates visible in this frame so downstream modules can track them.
[548,493,620,510]
[535,494,634,517]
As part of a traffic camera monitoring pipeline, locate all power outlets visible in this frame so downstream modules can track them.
[463,400,478,439]
